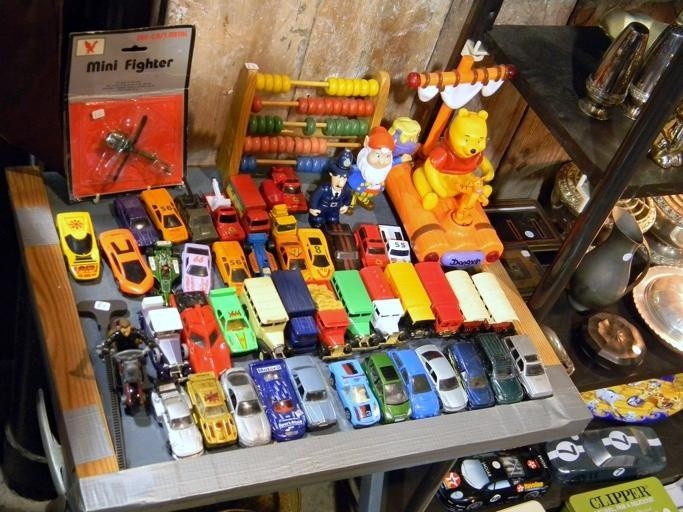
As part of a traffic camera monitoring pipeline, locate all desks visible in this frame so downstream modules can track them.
[5,163,594,512]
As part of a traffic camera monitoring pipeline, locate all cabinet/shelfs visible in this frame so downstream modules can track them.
[409,0,683,512]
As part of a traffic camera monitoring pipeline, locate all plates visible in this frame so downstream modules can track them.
[632,264,683,356]
